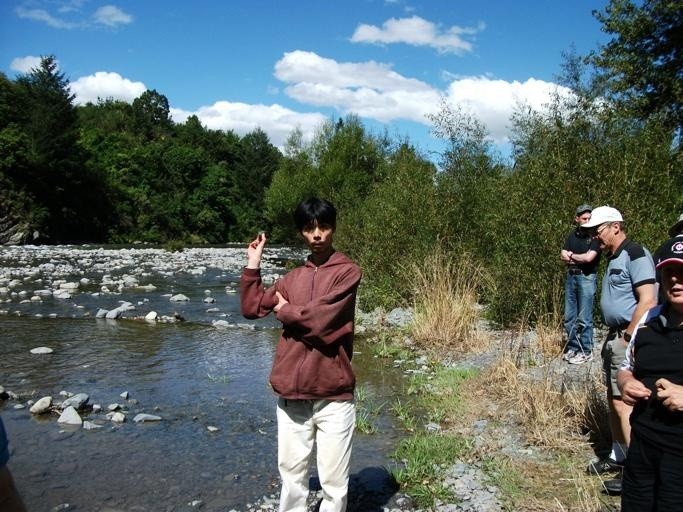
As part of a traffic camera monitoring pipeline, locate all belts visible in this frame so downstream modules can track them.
[607,321,630,334]
[569,268,584,275]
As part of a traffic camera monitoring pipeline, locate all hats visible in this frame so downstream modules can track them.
[668,213,683,233]
[576,204,592,215]
[580,206,622,230]
[653,237,683,266]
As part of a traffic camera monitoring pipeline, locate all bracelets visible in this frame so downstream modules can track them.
[569,253,573,258]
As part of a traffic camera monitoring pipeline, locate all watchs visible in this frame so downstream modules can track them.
[622,330,632,343]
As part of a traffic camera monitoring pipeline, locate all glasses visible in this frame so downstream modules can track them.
[589,225,608,237]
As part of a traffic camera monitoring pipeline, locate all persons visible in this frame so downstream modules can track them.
[616,237,682,512]
[239,196,361,511]
[558,204,601,366]
[577,205,662,494]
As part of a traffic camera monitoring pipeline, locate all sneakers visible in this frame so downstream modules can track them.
[562,349,576,361]
[586,455,623,475]
[602,478,622,494]
[569,354,594,364]
